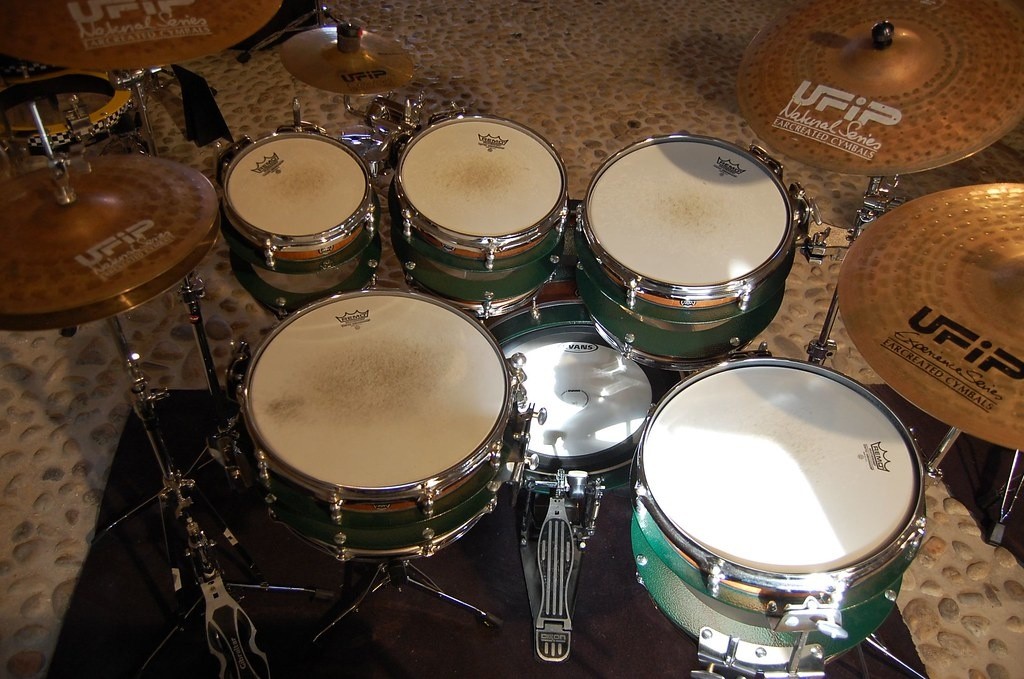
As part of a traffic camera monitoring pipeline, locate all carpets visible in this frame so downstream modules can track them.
[46,388,929,679]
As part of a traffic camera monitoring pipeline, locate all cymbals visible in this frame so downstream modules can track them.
[735,0,1022,181]
[281,27,415,96]
[0,1,283,71]
[0,155,220,331]
[834,182,1024,454]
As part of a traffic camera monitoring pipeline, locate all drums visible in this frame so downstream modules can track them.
[212,99,384,320]
[1,72,142,153]
[498,297,653,494]
[389,101,570,319]
[626,337,943,679]
[572,131,819,369]
[239,285,549,563]
